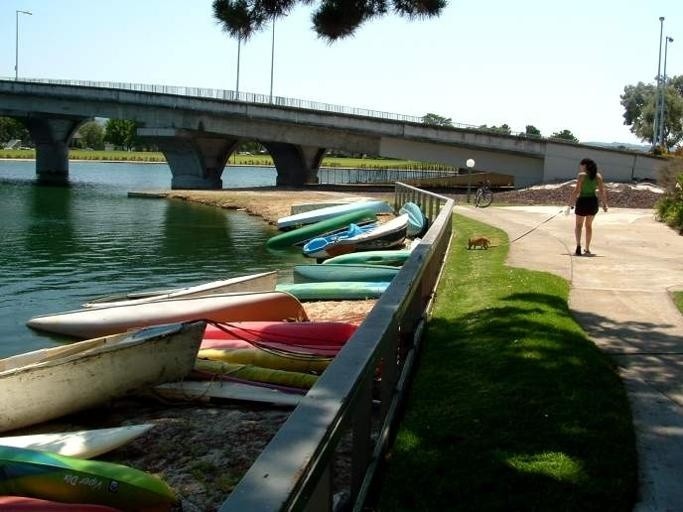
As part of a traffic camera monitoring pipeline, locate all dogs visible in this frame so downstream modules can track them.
[467,236,491,250]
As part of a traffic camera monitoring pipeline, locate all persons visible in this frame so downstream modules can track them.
[567,158,609,256]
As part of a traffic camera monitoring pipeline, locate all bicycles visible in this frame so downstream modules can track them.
[473,181,493,207]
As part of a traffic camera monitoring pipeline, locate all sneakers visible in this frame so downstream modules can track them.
[576,245,591,257]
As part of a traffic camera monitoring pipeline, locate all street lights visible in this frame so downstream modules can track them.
[653,17,673,153]
[466,158,474,203]
[15,11,31,81]
[270,12,287,104]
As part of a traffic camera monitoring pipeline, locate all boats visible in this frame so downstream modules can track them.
[0,321,207,434]
[0,423,180,510]
[267,200,425,301]
